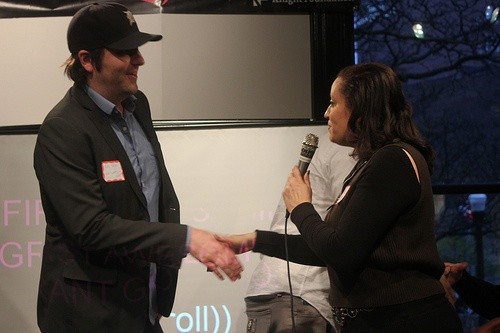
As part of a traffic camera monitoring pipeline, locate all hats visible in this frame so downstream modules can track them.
[67,3,162,52]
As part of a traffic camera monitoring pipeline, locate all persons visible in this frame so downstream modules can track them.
[207,63,463,333]
[33,2,244,333]
[244,132,467,333]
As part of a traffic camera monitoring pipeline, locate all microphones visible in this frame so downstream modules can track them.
[286,132,319,219]
